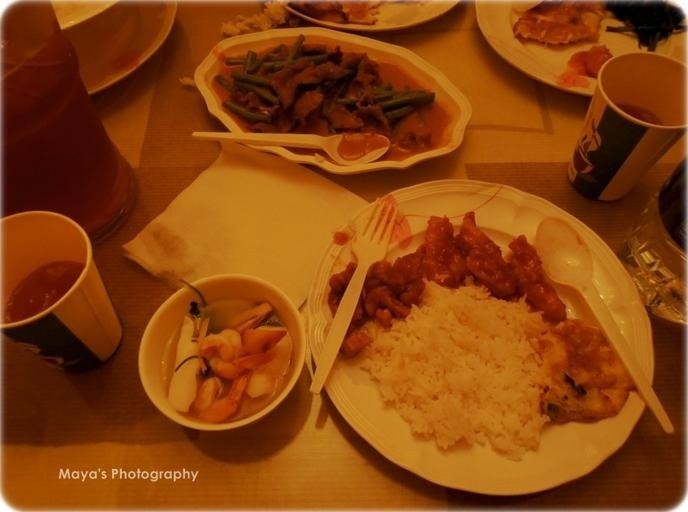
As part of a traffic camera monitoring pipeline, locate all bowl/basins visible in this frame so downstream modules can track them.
[137,272,308,435]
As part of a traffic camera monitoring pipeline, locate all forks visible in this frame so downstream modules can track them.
[309,193,403,399]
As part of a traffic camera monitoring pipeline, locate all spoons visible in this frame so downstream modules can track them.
[534,215,676,440]
[186,124,393,169]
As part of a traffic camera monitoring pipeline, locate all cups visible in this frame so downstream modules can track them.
[565,48,687,202]
[0,209,124,377]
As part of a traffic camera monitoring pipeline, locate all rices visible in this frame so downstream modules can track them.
[361,283,552,463]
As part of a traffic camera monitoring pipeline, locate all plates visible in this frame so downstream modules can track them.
[474,0,687,99]
[193,24,474,179]
[310,179,656,502]
[273,0,463,34]
[84,1,180,99]
